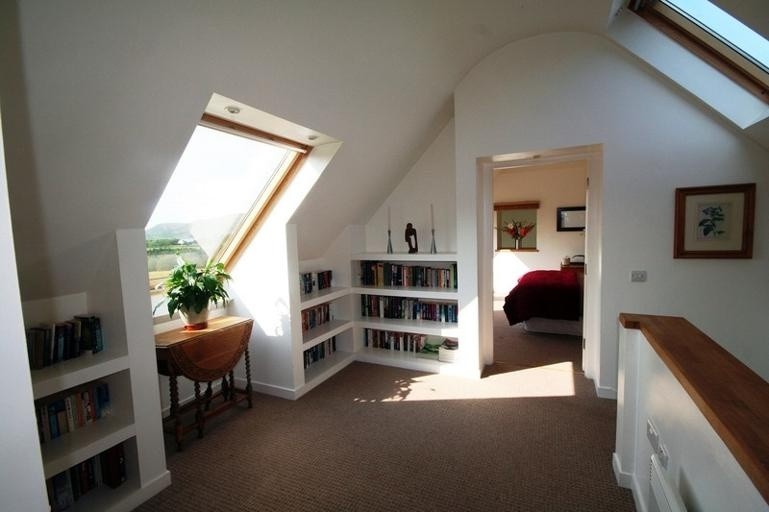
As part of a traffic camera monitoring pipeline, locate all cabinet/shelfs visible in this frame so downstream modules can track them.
[351,251,457,375]
[31,351,140,512]
[301,287,354,394]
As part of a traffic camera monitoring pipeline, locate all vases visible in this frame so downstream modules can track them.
[515,237,522,249]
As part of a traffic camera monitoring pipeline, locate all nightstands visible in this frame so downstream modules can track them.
[561,262,584,272]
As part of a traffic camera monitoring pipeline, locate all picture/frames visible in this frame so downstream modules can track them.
[674,183,756,260]
[557,206,586,231]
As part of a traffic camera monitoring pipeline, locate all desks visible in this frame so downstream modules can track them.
[154,316,254,452]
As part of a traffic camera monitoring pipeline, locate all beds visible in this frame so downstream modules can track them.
[503,270,582,338]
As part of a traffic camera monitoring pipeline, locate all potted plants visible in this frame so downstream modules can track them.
[152,258,232,330]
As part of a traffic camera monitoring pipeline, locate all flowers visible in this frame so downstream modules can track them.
[494,219,536,240]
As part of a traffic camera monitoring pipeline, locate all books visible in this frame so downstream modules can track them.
[361,260,458,363]
[25,316,128,512]
[300,271,336,369]
